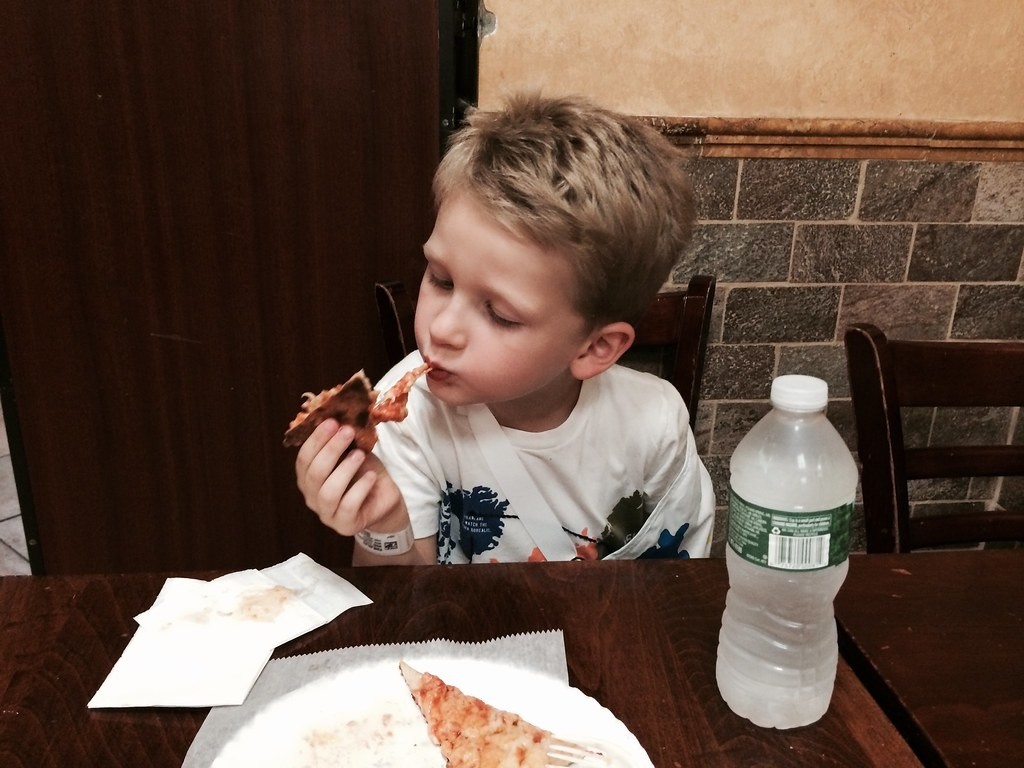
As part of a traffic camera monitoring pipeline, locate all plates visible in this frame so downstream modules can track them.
[210,656,655,768]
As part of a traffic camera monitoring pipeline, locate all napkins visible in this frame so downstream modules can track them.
[87,551,568,767]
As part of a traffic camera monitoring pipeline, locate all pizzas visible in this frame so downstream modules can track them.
[399,660,594,768]
[283,360,430,457]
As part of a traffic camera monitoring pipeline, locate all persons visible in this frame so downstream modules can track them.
[295,91,716,568]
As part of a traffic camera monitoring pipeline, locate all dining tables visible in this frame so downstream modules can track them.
[833,547,1024,768]
[0,557,926,768]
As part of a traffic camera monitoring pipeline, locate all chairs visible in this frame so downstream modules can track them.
[844,323,1023,556]
[375,275,718,431]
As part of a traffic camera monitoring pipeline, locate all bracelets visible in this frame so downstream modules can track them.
[353,521,413,557]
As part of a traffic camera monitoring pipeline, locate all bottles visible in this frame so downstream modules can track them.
[716,375,858,730]
